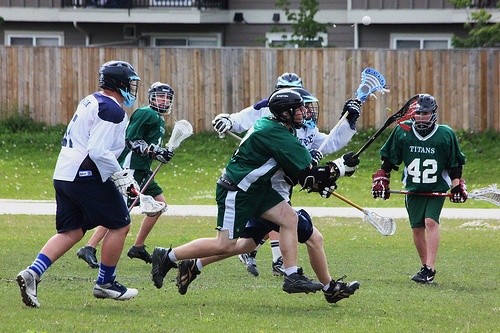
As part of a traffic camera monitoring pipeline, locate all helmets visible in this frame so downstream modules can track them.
[148,81,175,113]
[267,72,320,129]
[410,93,438,113]
[98,61,141,108]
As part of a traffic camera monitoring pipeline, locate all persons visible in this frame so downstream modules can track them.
[15,61,138,307]
[371,94,468,283]
[76,82,174,268]
[151,73,361,304]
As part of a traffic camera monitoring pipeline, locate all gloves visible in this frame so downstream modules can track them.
[309,148,323,170]
[340,98,361,121]
[371,169,392,200]
[127,184,138,200]
[148,143,175,164]
[317,180,338,199]
[326,151,361,178]
[211,114,233,134]
[449,185,468,204]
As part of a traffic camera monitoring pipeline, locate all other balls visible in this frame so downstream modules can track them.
[362,15,372,25]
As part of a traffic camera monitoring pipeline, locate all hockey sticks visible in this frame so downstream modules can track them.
[349,94,426,159]
[130,186,167,217]
[319,64,390,160]
[378,182,500,206]
[331,190,398,239]
[225,129,245,143]
[128,118,195,214]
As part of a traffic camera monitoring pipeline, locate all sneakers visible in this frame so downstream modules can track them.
[77,245,100,269]
[175,259,201,296]
[282,267,324,294]
[238,249,260,277]
[271,256,286,278]
[321,275,361,303]
[410,264,436,284]
[150,244,179,289]
[92,274,139,301]
[127,245,153,264]
[14,266,43,308]
